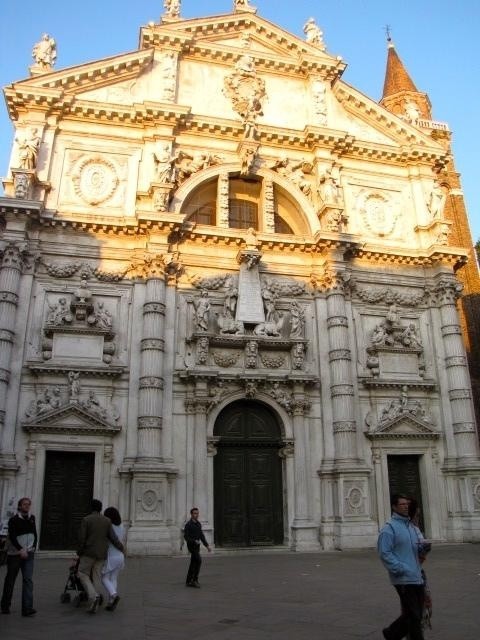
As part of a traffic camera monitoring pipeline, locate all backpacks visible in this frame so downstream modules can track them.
[3,531,12,553]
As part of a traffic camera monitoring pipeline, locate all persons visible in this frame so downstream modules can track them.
[191,290,211,331]
[35,387,62,415]
[242,103,262,140]
[47,297,67,326]
[246,256,257,270]
[224,276,238,315]
[400,386,409,407]
[274,155,312,196]
[94,302,112,327]
[414,399,426,417]
[271,383,294,417]
[320,166,338,203]
[289,300,305,339]
[205,381,226,413]
[184,507,212,588]
[0,498,38,616]
[245,377,262,399]
[85,390,106,421]
[155,142,172,183]
[406,499,433,634]
[164,0,181,18]
[101,505,124,611]
[405,323,422,350]
[31,32,57,69]
[178,150,208,178]
[376,492,428,640]
[244,147,257,170]
[262,280,278,322]
[15,126,41,168]
[427,181,445,220]
[381,398,398,424]
[70,373,79,396]
[304,17,324,46]
[72,499,124,613]
[404,99,421,129]
[75,274,91,303]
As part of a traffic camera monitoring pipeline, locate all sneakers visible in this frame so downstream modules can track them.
[187,580,202,588]
[381,627,400,640]
[0,603,11,616]
[21,608,37,617]
[104,594,120,612]
[85,593,103,615]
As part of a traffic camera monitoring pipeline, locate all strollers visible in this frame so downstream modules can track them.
[60,556,102,608]
